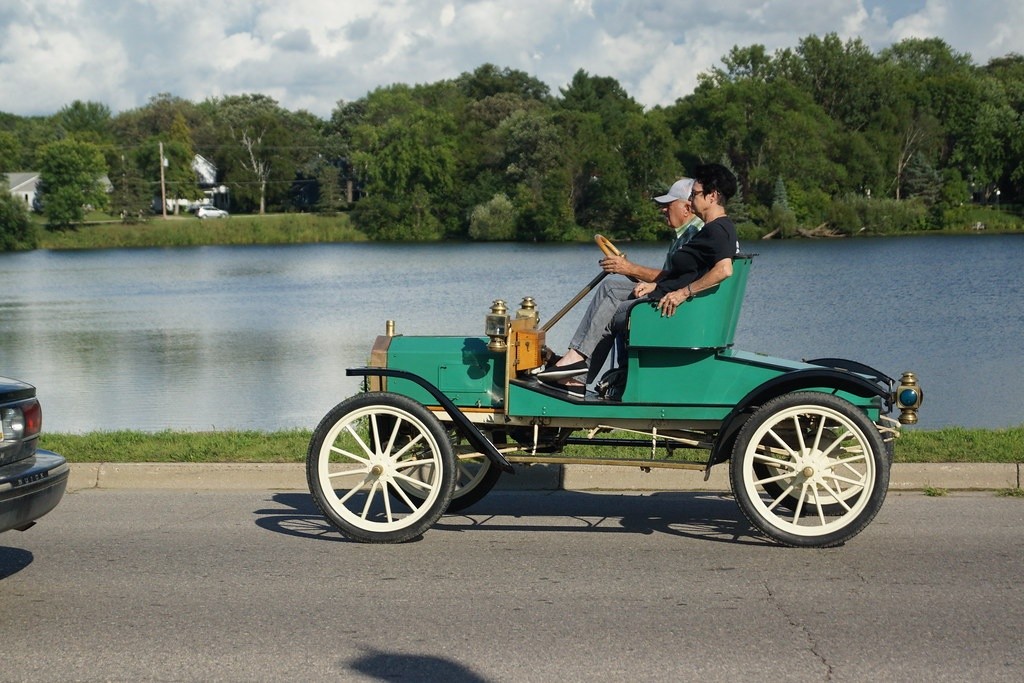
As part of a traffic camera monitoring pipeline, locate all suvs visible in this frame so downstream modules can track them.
[195,205,228,219]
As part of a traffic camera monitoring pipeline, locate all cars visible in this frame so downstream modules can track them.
[305,234,923,549]
[0,376,70,534]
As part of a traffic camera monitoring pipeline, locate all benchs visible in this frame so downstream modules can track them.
[629,253,756,350]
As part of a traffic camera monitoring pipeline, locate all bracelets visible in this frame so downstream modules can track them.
[688,284,694,295]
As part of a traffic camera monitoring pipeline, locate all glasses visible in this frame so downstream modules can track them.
[691,190,721,198]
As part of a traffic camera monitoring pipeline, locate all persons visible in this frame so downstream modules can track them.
[536,163,739,398]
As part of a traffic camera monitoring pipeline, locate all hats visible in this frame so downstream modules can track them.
[654,179,697,203]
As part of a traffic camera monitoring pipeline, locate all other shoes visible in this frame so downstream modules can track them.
[537,360,589,383]
[537,375,586,398]
